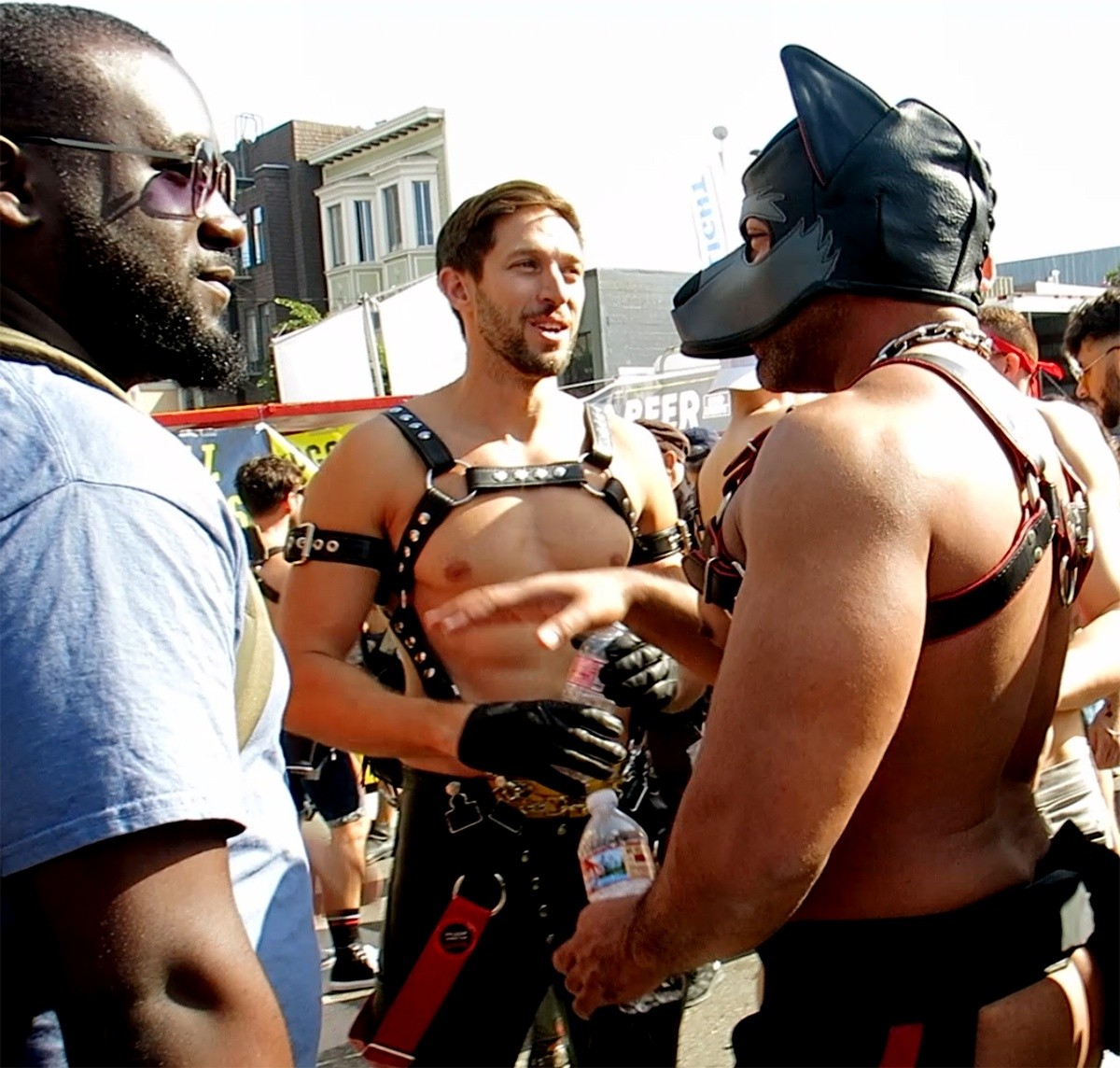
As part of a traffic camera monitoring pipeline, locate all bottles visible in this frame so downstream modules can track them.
[562,623,634,784]
[579,789,685,1013]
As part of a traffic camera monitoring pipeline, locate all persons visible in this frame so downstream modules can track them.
[1,3,1120,1068]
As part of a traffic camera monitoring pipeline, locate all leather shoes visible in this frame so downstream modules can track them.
[364,820,395,865]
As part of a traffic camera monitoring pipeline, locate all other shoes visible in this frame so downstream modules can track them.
[528,1041,569,1068]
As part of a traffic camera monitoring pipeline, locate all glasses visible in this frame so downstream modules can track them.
[1074,346,1120,381]
[7,137,235,220]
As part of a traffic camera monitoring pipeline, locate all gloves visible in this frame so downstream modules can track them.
[458,699,627,799]
[571,630,677,712]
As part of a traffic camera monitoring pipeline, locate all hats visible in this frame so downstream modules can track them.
[680,428,718,462]
[671,44,998,359]
[633,418,688,464]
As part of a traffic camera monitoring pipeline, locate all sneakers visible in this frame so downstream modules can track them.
[328,942,378,990]
[684,960,724,1012]
